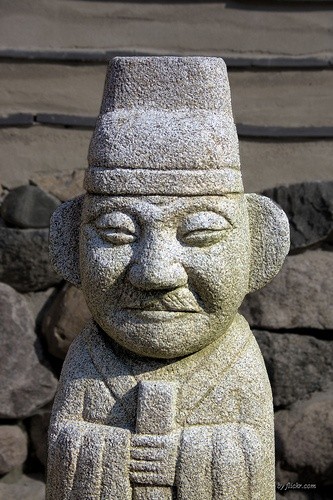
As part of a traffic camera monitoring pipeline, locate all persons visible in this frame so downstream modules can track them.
[39,54,296,499]
